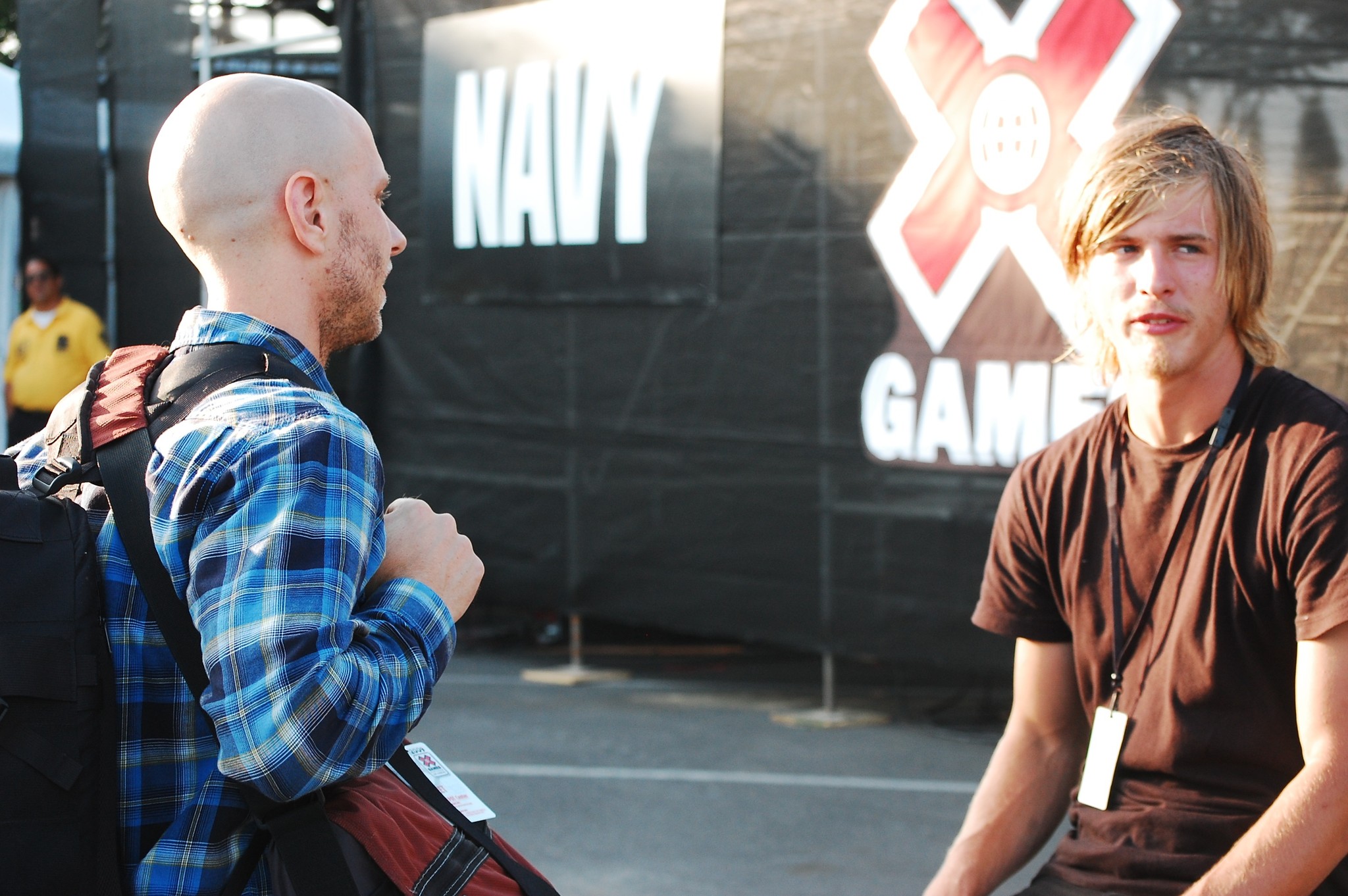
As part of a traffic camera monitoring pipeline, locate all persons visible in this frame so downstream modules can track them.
[5,75,483,896]
[921,104,1348,896]
[5,251,111,444]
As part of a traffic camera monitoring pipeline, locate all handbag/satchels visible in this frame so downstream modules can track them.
[261,755,559,896]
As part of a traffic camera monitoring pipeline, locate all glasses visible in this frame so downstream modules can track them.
[24,272,54,284]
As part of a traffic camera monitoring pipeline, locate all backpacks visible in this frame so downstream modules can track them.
[1,353,338,896]
